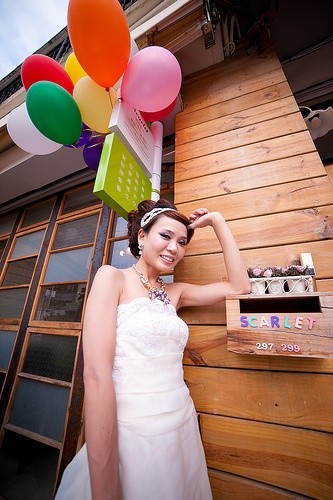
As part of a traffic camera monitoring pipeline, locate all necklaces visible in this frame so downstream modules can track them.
[133,261,171,305]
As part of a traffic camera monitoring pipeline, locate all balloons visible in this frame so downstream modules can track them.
[7,0,182,168]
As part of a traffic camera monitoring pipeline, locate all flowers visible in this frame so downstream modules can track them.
[248,265,308,278]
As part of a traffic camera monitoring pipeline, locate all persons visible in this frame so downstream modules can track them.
[56,199,253,500]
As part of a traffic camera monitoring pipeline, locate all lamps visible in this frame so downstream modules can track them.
[299,106,333,140]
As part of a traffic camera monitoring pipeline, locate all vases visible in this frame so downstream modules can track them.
[251,280,266,294]
[266,280,285,293]
[287,279,306,292]
